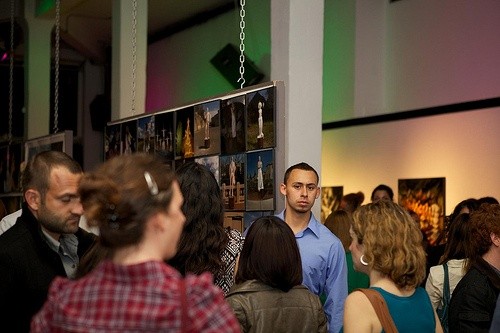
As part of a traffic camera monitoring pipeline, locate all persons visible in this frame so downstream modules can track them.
[30,152,242,333]
[340,191,364,211]
[324,208,371,294]
[372,184,395,203]
[0,149,99,333]
[242,163,348,333]
[410,196,500,333]
[343,202,443,333]
[225,216,327,333]
[164,161,245,297]
